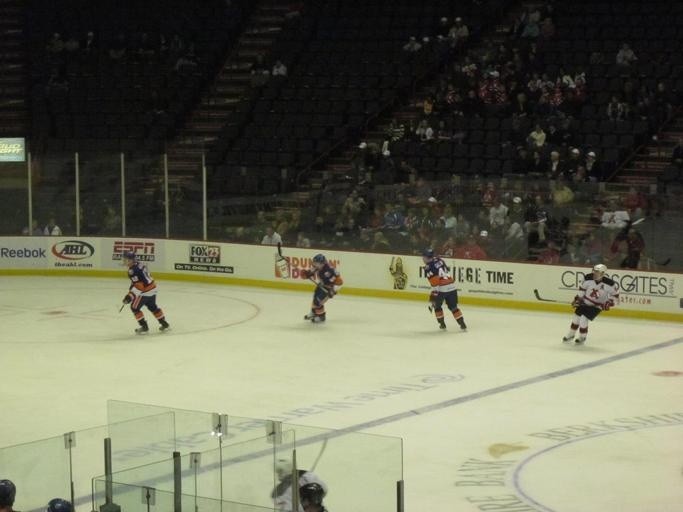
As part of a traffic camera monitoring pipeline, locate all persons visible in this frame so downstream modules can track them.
[47,30,98,63]
[237,208,323,248]
[269,454,328,512]
[22,216,63,236]
[316,2,673,268]
[249,53,288,80]
[71,204,121,234]
[562,263,621,344]
[0,478,21,512]
[122,250,170,334]
[302,253,344,321]
[133,32,196,65]
[421,248,467,330]
[45,498,73,512]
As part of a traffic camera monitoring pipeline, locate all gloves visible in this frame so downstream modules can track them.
[328,288,336,298]
[122,291,136,303]
[572,296,583,307]
[602,300,614,310]
[301,269,312,279]
[428,291,438,302]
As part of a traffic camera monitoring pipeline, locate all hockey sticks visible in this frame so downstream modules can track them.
[311,435,327,471]
[534,289,602,310]
[428,304,434,312]
[118,290,130,312]
[277,242,329,293]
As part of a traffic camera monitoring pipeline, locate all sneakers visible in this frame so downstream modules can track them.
[135,320,149,332]
[312,313,325,323]
[437,317,446,329]
[458,318,466,329]
[304,308,316,319]
[159,320,169,330]
[563,332,575,341]
[575,335,586,343]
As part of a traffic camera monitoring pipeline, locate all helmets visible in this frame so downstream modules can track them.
[275,456,294,477]
[422,247,434,257]
[123,250,136,260]
[313,254,325,263]
[593,263,607,273]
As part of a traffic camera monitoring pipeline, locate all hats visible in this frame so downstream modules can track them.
[428,196,437,203]
[358,142,367,148]
[489,70,499,77]
[572,148,579,154]
[480,230,488,236]
[382,150,390,156]
[588,151,596,157]
[512,196,522,204]
